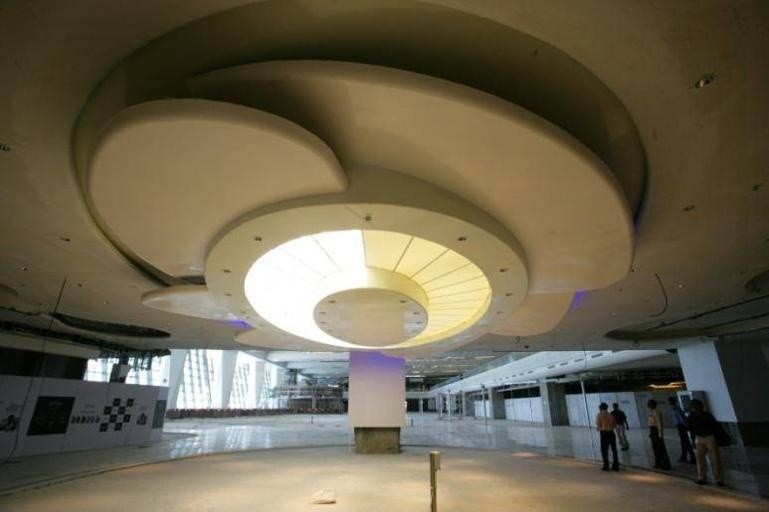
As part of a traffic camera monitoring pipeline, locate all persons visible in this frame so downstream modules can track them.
[610,403,630,451]
[646,399,673,470]
[596,403,620,472]
[686,400,696,450]
[668,396,696,464]
[686,398,726,487]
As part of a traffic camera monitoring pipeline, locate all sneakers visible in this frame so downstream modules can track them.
[694,478,723,487]
